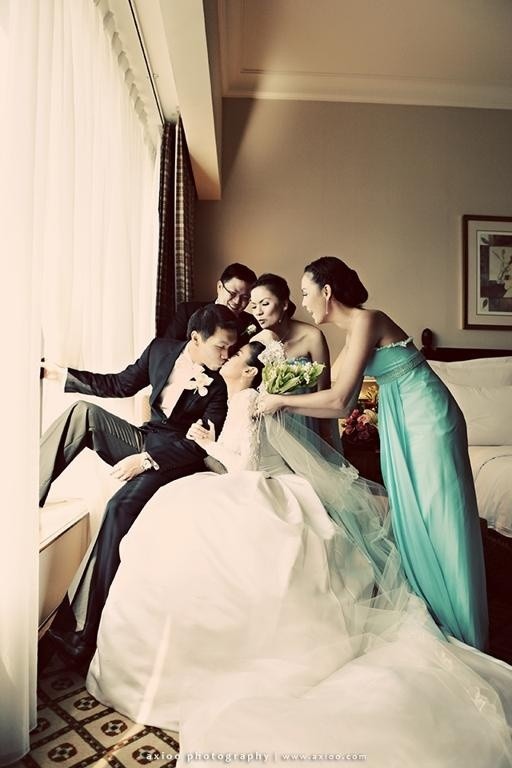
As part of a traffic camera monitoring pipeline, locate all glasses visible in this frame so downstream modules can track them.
[223,286,251,304]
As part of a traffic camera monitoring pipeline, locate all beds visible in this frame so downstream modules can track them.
[419,328,512,669]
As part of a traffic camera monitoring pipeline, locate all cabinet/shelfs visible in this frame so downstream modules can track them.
[331,379,385,492]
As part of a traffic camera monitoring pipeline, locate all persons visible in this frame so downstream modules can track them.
[246,252,493,652]
[165,261,261,362]
[247,271,347,481]
[36,300,238,677]
[82,328,380,731]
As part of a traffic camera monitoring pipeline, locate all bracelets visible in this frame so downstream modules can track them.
[140,449,152,472]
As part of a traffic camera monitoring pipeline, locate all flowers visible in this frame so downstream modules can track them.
[253,343,379,446]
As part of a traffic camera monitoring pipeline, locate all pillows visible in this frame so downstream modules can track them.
[443,382,512,448]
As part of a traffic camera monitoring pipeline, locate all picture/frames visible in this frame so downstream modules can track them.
[461,214,512,333]
